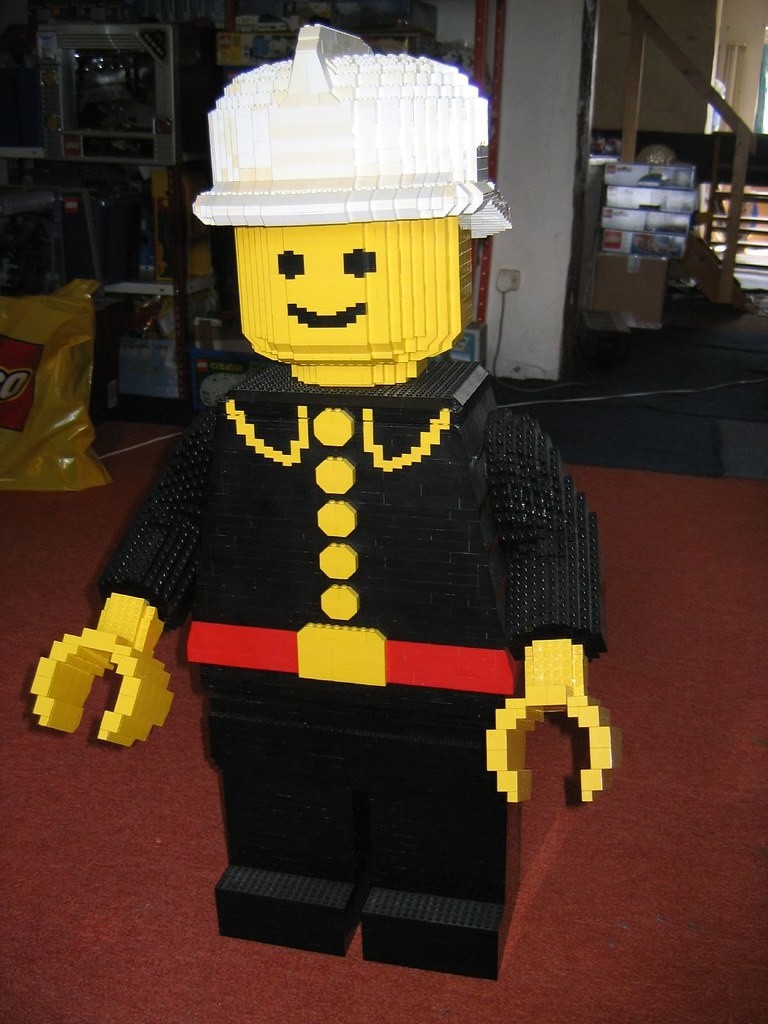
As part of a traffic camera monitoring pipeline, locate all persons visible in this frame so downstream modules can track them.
[28,25,614,980]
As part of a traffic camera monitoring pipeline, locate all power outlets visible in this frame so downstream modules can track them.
[501,270,522,292]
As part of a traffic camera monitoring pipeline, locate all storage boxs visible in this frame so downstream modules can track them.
[579,166,699,330]
